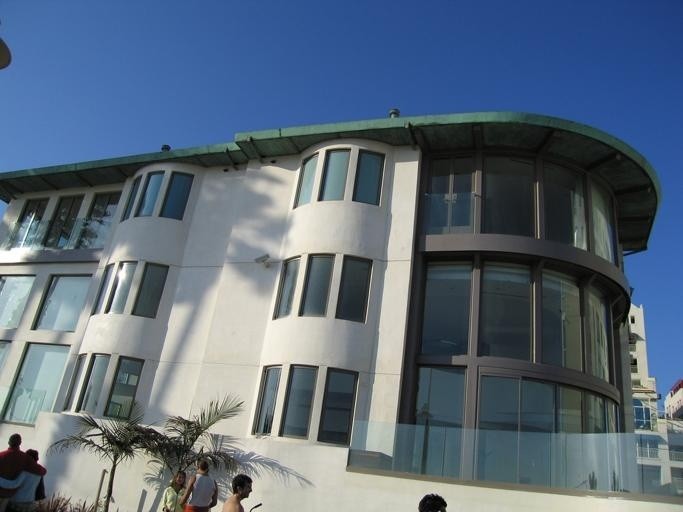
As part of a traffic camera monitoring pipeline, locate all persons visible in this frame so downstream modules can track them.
[177,461,218,512]
[0,433,46,512]
[0,448,42,504]
[418,493,447,512]
[220,474,252,512]
[162,469,187,512]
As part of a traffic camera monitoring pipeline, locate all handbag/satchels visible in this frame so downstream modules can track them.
[35,479,46,501]
